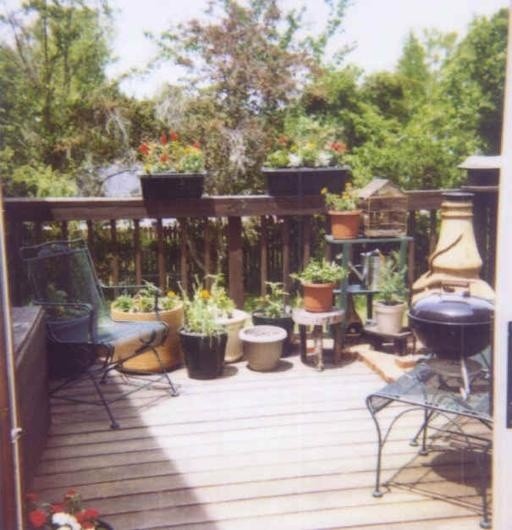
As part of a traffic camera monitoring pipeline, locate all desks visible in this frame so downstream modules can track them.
[323,234,415,349]
[365,345,494,529]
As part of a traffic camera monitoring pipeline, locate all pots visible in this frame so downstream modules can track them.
[407,293,494,359]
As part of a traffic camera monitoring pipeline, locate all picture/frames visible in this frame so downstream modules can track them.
[261,170,347,195]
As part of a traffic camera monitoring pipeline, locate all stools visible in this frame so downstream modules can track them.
[364,322,412,356]
[292,308,345,363]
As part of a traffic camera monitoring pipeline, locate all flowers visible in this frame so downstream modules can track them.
[264,134,346,168]
[320,182,358,211]
[199,286,236,320]
[134,128,205,175]
[110,290,175,313]
[24,282,81,322]
[24,489,101,529]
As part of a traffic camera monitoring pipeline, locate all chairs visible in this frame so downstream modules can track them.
[17,236,180,429]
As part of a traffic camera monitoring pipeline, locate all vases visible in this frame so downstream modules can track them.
[93,516,111,528]
[327,209,362,240]
[109,298,183,375]
[206,309,246,363]
[24,304,92,380]
[140,175,205,200]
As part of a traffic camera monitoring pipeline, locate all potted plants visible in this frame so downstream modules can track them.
[288,254,350,312]
[176,273,228,379]
[371,249,409,333]
[243,281,295,357]
